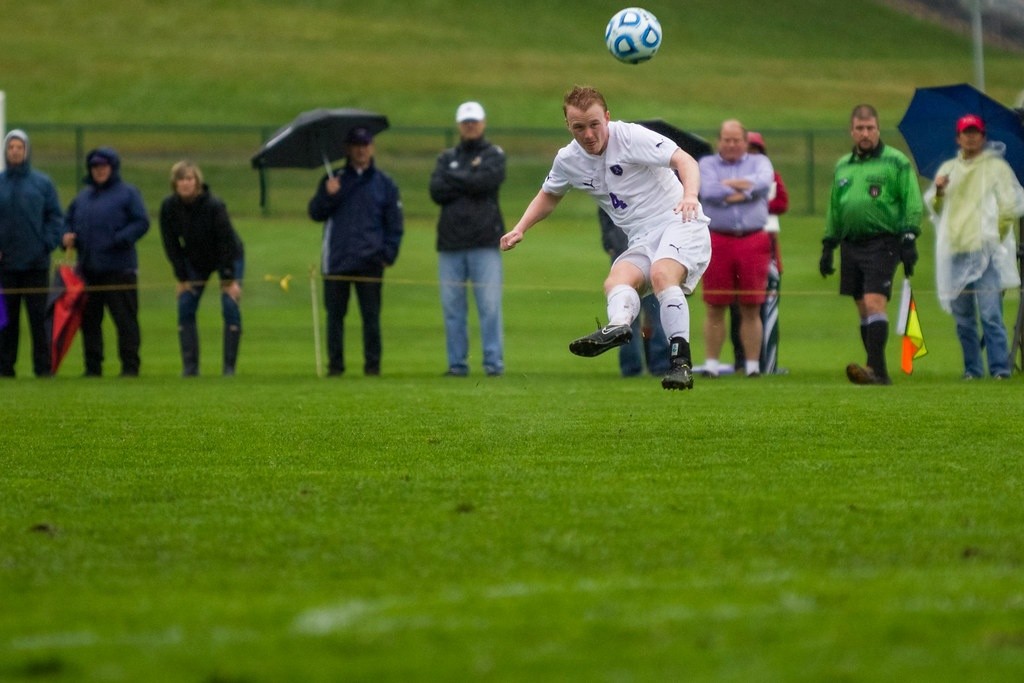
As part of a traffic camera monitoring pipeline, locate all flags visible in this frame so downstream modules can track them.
[897,280,928,376]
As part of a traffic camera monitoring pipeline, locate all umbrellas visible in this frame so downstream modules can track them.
[630,119,714,162]
[48,247,89,376]
[250,107,392,178]
[898,82,1024,189]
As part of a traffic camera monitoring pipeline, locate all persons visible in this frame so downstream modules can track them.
[428,100,507,377]
[59,146,151,378]
[924,115,1024,380]
[597,206,671,377]
[730,131,788,376]
[697,120,773,377]
[307,126,405,375]
[499,86,712,390]
[157,161,245,376]
[820,104,924,386]
[0,129,63,377]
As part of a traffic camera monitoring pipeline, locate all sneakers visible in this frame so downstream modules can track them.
[661,357,694,390]
[569,323,632,357]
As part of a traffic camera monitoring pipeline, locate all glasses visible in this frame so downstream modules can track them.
[351,141,370,146]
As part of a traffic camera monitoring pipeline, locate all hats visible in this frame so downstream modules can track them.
[345,127,371,143]
[456,101,485,123]
[89,156,106,166]
[747,131,766,154]
[957,113,985,134]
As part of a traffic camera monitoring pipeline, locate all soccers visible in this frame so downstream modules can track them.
[603,6,663,66]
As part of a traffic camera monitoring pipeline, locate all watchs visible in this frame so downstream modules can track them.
[904,233,915,241]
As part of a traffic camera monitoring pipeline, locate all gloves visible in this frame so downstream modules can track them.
[902,236,918,279]
[820,242,836,277]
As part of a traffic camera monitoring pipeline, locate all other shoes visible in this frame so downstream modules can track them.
[845,361,879,384]
[699,365,718,378]
[963,372,979,378]
[747,372,759,378]
[996,375,1009,378]
[445,370,456,375]
